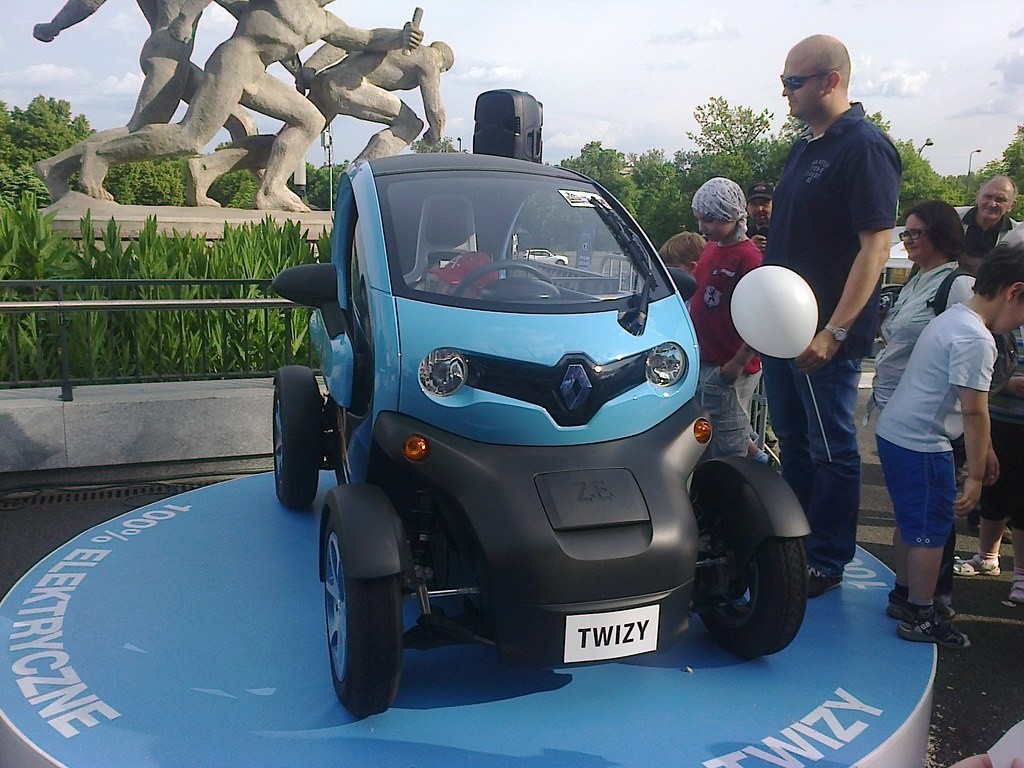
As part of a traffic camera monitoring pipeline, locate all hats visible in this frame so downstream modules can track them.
[747,182,776,200]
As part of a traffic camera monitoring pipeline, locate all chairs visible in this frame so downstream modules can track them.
[400,194,476,293]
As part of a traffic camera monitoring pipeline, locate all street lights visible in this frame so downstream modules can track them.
[968,149,982,174]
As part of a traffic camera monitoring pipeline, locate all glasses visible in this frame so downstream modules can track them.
[780,72,829,89]
[899,229,930,241]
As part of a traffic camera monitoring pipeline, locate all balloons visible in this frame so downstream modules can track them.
[731,266,819,358]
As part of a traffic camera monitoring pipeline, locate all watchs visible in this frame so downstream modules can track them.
[824,323,849,343]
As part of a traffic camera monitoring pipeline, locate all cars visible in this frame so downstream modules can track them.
[273,152,814,718]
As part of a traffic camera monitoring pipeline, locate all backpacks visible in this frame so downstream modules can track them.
[934,268,1018,390]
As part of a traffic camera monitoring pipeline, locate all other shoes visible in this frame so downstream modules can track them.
[806,565,841,596]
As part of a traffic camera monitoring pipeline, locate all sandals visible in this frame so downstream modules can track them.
[897,608,966,648]
[1009,576,1024,602]
[887,589,956,620]
[952,555,1000,576]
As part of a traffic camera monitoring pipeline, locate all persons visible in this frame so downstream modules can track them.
[858,174,1023,606]
[656,177,782,470]
[757,37,900,599]
[29,0,266,213]
[77,0,424,215]
[179,28,456,206]
[873,240,1024,648]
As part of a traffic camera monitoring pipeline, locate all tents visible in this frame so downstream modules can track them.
[881,242,915,284]
